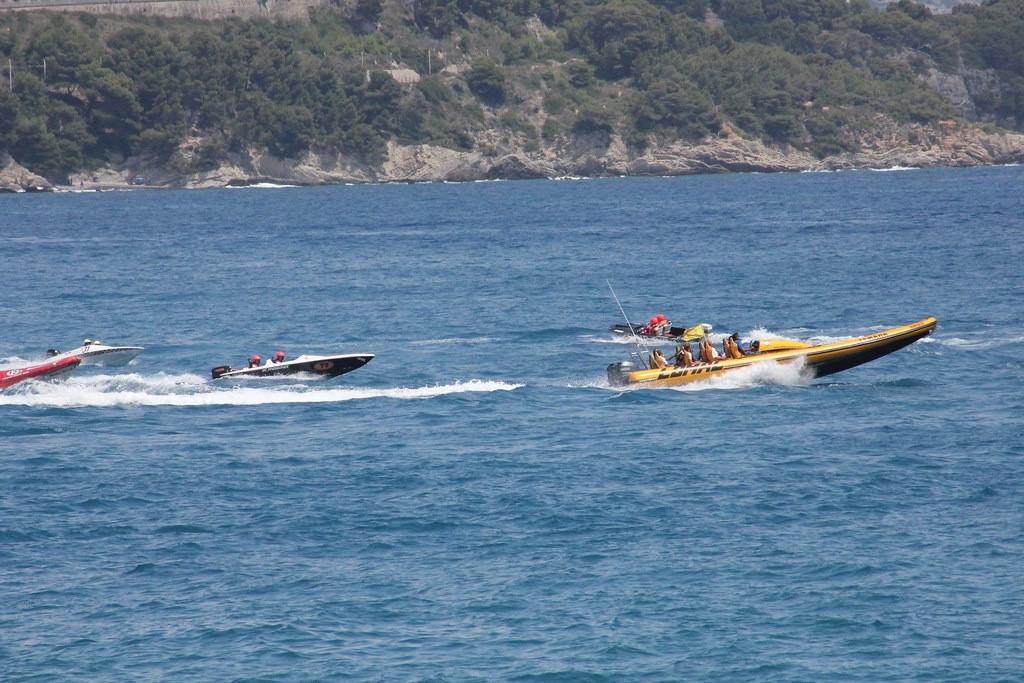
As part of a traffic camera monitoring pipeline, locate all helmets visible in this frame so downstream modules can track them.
[656,314,665,322]
[653,348,663,357]
[94,341,101,345]
[277,352,284,360]
[252,355,261,363]
[650,318,658,324]
[83,339,91,346]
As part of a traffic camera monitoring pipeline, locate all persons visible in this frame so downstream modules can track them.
[274,352,284,364]
[702,338,727,360]
[83,339,91,346]
[728,332,754,355]
[653,348,675,367]
[679,342,699,364]
[252,354,261,367]
[641,314,664,335]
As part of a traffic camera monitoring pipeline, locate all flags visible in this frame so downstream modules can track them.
[683,324,704,341]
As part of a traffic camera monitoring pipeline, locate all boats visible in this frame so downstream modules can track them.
[607,323,713,344]
[42,345,146,367]
[210,353,376,382]
[0,355,82,388]
[605,316,937,388]
